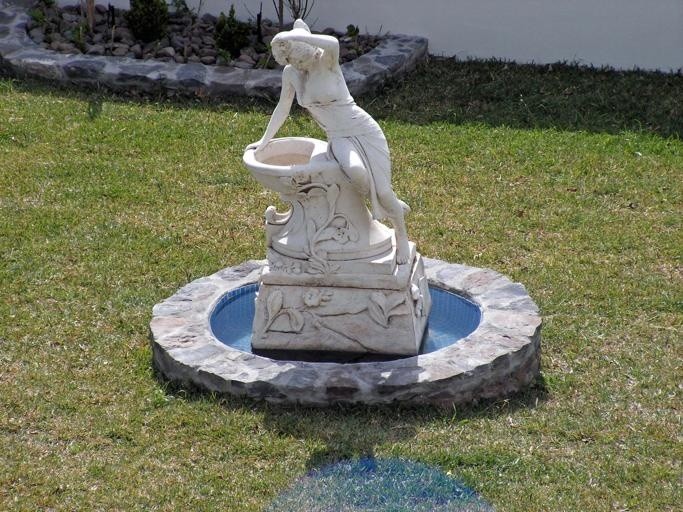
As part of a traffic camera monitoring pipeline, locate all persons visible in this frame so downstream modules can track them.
[243,19,411,264]
